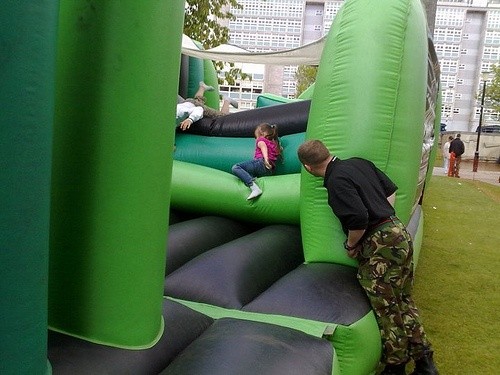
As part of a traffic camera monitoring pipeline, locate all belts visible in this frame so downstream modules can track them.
[360,216,398,240]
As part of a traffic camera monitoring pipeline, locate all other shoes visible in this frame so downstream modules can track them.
[410,354,439,375]
[448,173,460,178]
[379,351,407,375]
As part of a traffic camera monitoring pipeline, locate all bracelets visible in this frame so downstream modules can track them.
[344,239,353,250]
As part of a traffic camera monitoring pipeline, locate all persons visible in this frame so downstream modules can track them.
[444,134,464,177]
[175,81,238,132]
[231,122,281,200]
[297,140,438,375]
